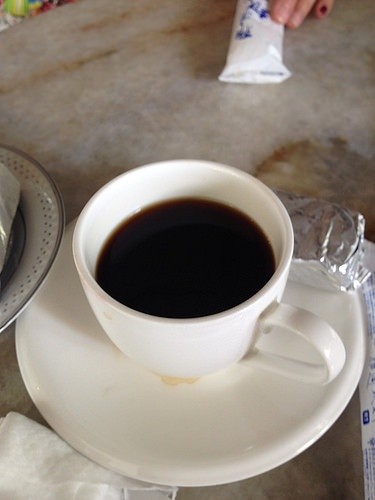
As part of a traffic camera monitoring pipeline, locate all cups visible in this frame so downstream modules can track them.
[73,156,347,388]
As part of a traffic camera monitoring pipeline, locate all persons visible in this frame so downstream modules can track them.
[268,0,335,29]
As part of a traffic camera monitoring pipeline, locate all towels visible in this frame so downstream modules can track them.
[0,409,179,500]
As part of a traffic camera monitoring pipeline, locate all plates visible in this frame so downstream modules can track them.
[0,139,65,335]
[14,197,368,488]
